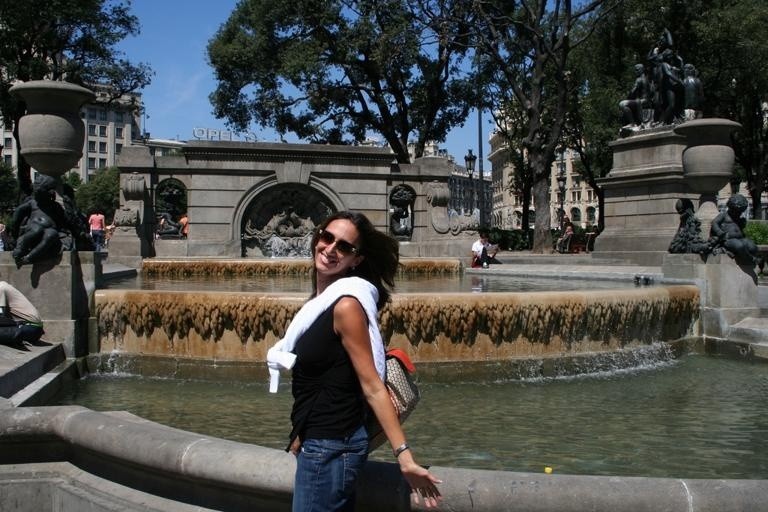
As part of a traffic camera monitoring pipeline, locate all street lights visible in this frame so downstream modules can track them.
[464,150,476,214]
[557,174,567,230]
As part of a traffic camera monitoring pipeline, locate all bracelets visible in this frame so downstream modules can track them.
[393,444,408,455]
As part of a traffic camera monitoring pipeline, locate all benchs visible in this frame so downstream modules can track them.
[558,232,584,255]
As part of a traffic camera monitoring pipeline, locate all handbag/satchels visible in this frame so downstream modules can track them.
[358,348,421,454]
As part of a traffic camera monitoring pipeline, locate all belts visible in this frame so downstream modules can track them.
[18,323,43,328]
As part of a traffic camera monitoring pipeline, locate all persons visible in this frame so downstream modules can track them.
[11,172,66,264]
[667,198,706,254]
[0,279,43,346]
[268,211,445,511]
[88,209,107,251]
[711,194,758,265]
[180,213,188,237]
[556,216,573,249]
[591,225,599,238]
[0,221,6,250]
[471,233,502,268]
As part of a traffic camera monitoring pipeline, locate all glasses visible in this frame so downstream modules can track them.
[317,228,360,255]
